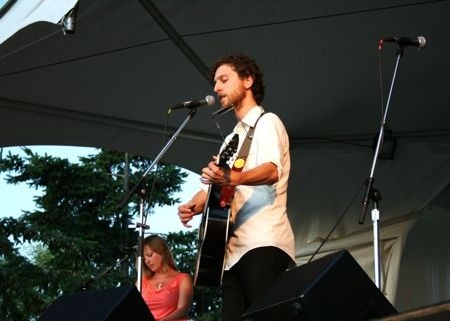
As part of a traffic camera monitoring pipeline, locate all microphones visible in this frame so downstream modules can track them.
[383,36,426,49]
[172,95,216,110]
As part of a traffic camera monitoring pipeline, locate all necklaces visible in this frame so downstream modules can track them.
[159,283,161,288]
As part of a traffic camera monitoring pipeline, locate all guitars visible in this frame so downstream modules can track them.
[194,134,240,289]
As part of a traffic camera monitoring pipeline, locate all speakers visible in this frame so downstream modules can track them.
[38,286,157,321]
[242,248,400,321]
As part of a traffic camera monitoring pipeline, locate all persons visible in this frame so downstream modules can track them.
[178,53,298,321]
[141,235,194,321]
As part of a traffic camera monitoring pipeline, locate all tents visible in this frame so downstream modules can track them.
[0,0,450,313]
